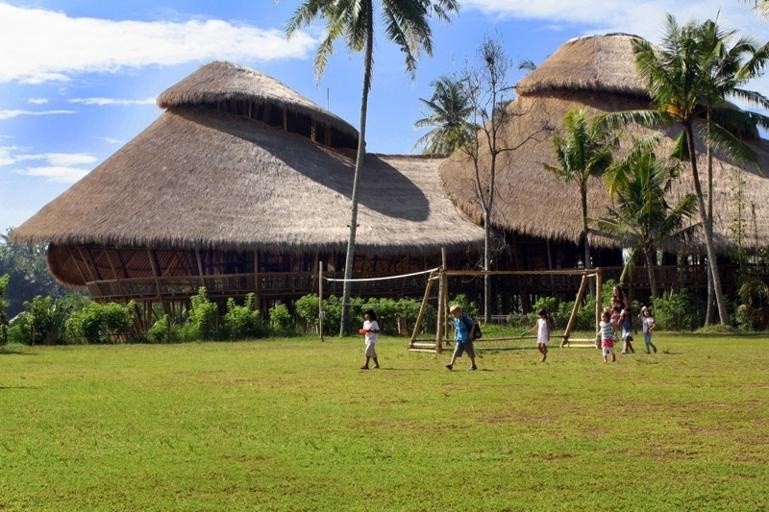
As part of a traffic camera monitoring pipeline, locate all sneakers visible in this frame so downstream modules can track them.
[446,365,453,370]
[653,347,656,352]
[621,351,627,354]
[631,351,635,353]
[470,365,477,370]
[645,352,650,353]
[374,366,379,368]
[360,367,369,369]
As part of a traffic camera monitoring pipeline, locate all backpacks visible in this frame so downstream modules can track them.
[462,317,481,340]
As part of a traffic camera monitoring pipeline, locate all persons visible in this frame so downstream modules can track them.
[612,283,628,343]
[636,304,658,354]
[358,308,382,370]
[443,304,479,371]
[608,304,622,330]
[527,308,551,362]
[616,306,635,355]
[596,311,617,363]
